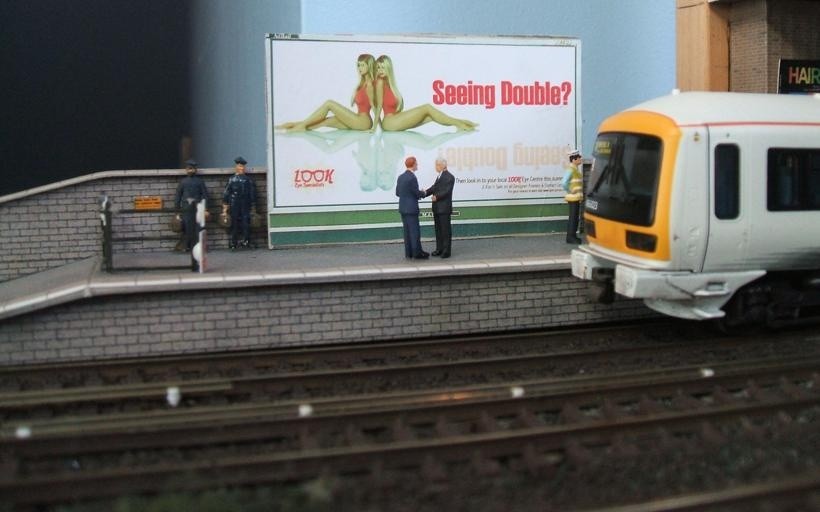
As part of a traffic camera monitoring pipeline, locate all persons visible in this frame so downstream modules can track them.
[379,132,469,189]
[392,156,434,262]
[562,149,587,246]
[171,160,212,251]
[293,135,381,194]
[277,54,385,133]
[367,55,480,134]
[221,157,260,251]
[423,157,455,260]
